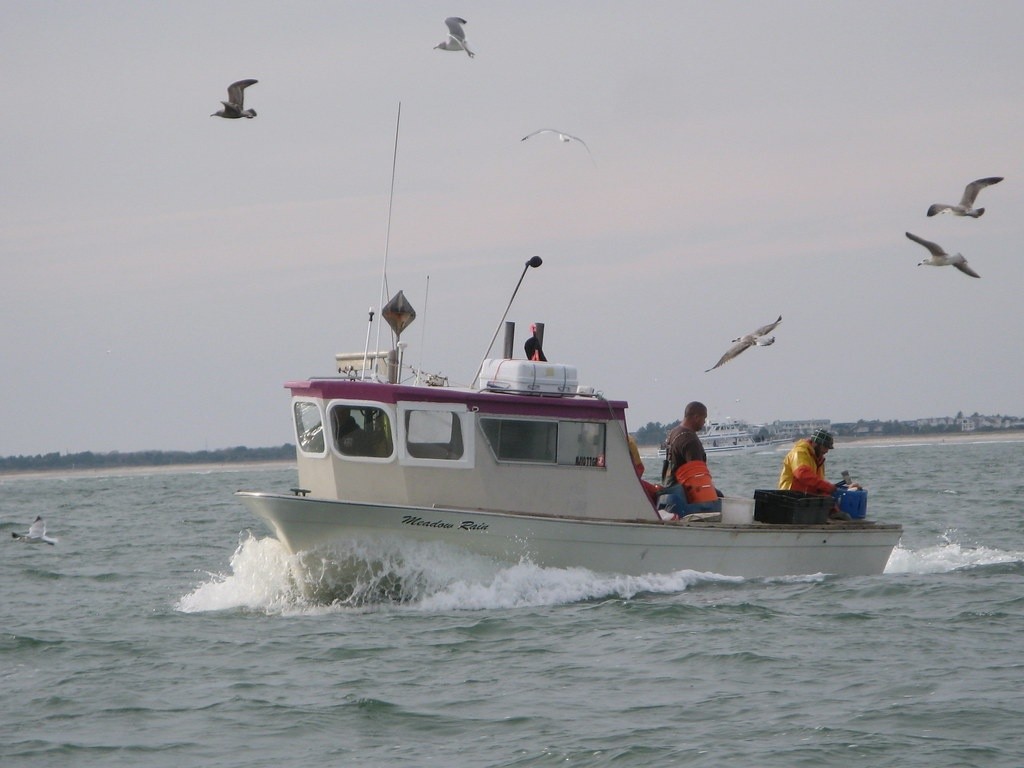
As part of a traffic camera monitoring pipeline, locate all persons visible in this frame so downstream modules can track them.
[304,405,356,459]
[662,401,724,498]
[778,429,858,513]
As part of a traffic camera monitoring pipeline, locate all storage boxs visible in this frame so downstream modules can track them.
[754,490,836,524]
[720,497,755,524]
[480,359,579,398]
[836,488,868,518]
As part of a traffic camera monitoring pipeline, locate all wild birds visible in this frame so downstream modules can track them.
[520,126,592,158]
[524,323,548,362]
[209,78,260,124]
[926,176,1002,222]
[706,315,783,376]
[901,230,981,280]
[10,513,60,549]
[432,14,485,58]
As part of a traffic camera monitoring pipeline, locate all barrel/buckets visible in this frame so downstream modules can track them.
[832,485,867,519]
[721,497,755,523]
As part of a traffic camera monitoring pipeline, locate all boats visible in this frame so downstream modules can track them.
[657,418,797,459]
[229,100,908,581]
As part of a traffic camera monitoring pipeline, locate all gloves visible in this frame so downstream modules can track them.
[826,482,836,493]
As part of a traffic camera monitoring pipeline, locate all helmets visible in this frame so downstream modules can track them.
[810,428,834,450]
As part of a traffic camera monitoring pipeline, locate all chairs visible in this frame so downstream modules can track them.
[666,484,720,517]
